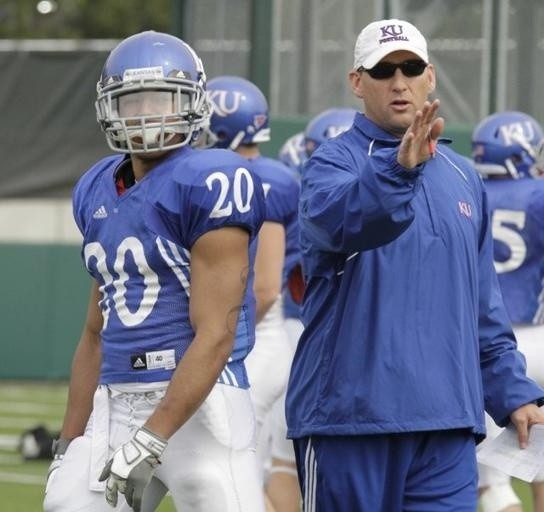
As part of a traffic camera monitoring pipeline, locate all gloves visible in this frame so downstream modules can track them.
[96,425,169,512]
[45,431,85,495]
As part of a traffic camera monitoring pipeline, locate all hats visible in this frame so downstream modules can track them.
[353,18,430,73]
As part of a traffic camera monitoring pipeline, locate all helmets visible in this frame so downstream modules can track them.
[199,74,273,150]
[304,107,363,158]
[277,129,312,177]
[470,109,544,180]
[95,29,207,98]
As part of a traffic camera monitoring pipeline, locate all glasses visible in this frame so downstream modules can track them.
[358,58,428,80]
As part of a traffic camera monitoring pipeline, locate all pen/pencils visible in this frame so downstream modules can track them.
[427,133,435,159]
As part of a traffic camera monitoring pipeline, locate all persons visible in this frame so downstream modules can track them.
[41,29,268,512]
[199,75,297,512]
[264,108,359,512]
[277,133,308,177]
[285,13,544,512]
[469,109,544,512]
